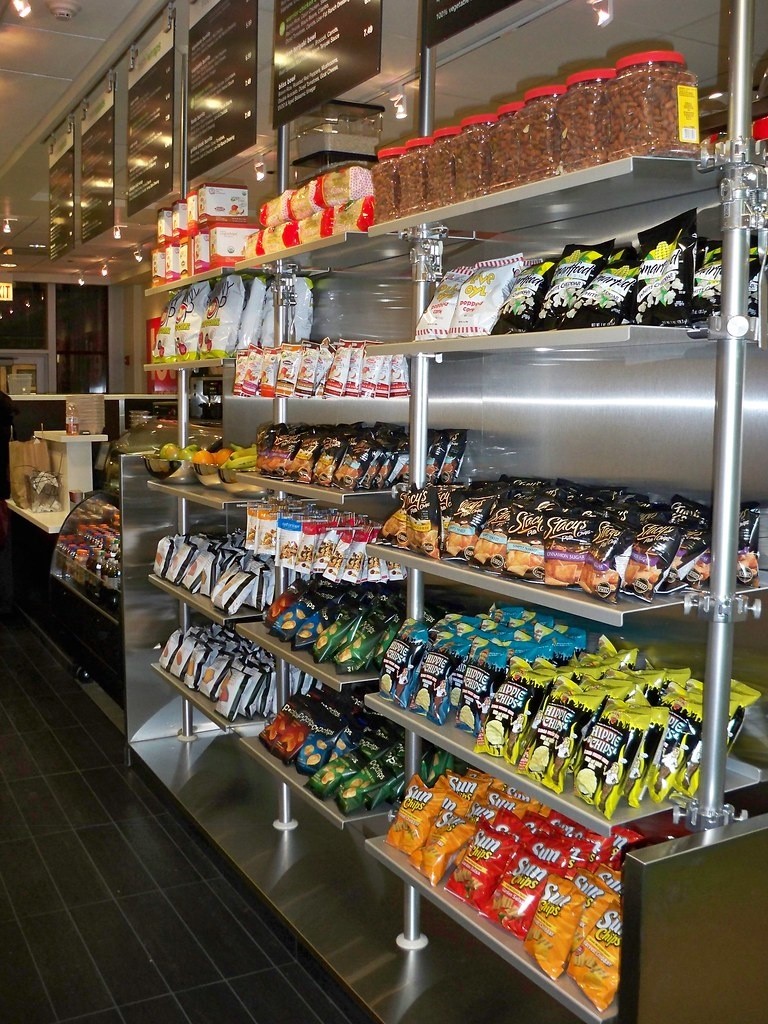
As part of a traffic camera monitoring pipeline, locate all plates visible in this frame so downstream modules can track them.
[129,410,150,428]
[65,394,106,435]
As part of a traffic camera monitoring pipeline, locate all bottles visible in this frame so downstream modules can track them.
[66,402,80,435]
[59,515,121,591]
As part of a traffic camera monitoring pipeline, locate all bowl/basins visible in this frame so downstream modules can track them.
[142,453,200,483]
[191,461,228,491]
[216,467,275,499]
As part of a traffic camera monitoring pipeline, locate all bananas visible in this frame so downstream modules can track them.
[218,442,257,472]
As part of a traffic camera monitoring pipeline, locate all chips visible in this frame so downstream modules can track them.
[381,673,598,797]
[383,517,757,595]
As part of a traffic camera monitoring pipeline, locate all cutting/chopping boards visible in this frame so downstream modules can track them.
[8,439,52,507]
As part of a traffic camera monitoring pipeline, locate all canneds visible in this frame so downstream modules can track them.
[369,50,699,225]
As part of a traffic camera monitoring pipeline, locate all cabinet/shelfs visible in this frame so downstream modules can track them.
[365,155,768,1022]
[234,230,418,834]
[146,266,333,743]
[5,431,109,611]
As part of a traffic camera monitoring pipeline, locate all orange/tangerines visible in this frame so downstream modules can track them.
[193,447,234,467]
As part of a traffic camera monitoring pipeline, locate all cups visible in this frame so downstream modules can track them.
[7,373,33,395]
